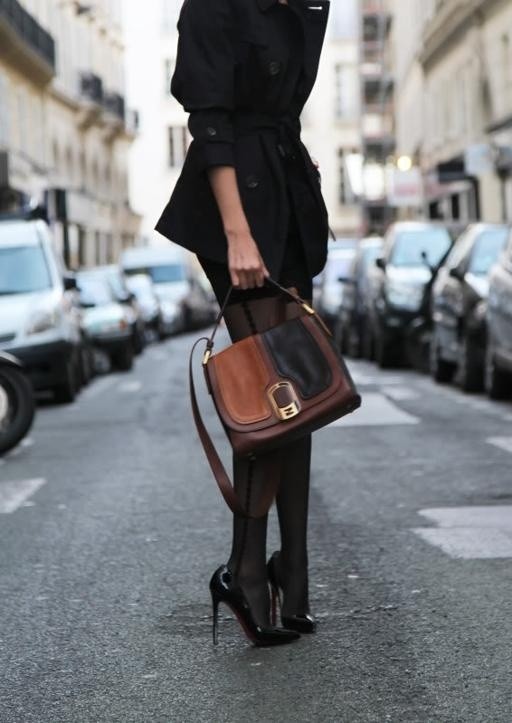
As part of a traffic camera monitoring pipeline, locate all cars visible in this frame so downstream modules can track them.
[312,217,512,398]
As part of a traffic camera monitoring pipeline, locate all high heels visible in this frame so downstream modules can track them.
[208,564,301,646]
[266,550,317,634]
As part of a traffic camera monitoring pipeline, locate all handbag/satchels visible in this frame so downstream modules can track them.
[200,273,361,458]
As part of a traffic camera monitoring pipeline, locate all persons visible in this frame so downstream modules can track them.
[154,0,330,645]
